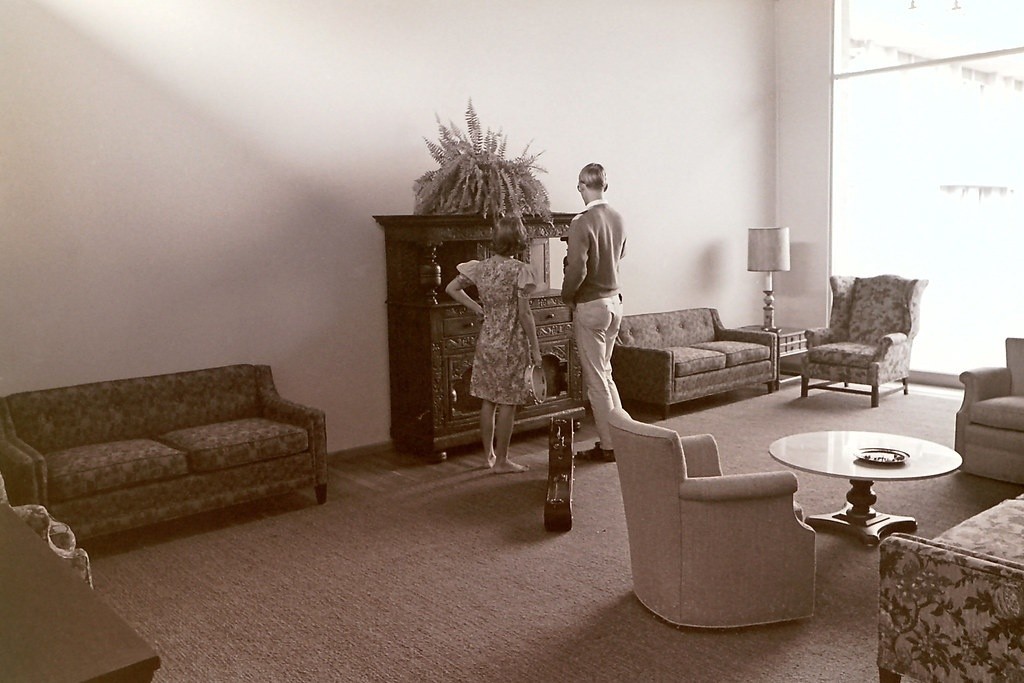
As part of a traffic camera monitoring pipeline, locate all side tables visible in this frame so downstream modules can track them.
[737,325,809,391]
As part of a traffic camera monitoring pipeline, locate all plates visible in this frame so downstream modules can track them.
[853,448,910,463]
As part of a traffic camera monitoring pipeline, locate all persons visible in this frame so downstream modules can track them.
[445,216,542,473]
[560,164,622,462]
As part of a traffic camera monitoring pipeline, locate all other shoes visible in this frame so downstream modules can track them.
[577,441,615,462]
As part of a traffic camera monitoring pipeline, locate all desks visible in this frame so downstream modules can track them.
[768,431,962,547]
[0,497,161,683]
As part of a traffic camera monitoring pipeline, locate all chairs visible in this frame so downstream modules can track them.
[800,275,929,408]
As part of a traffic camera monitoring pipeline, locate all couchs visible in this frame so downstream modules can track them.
[610,308,778,420]
[607,408,817,629]
[0,363,328,541]
[954,338,1024,484]
[877,489,1024,683]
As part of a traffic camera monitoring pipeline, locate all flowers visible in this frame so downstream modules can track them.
[413,98,555,231]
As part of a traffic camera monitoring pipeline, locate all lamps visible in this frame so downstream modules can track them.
[747,227,790,332]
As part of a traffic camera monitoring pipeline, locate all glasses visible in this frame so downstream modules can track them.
[577,186,582,192]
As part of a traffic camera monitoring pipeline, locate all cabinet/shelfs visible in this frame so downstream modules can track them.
[374,212,585,462]
